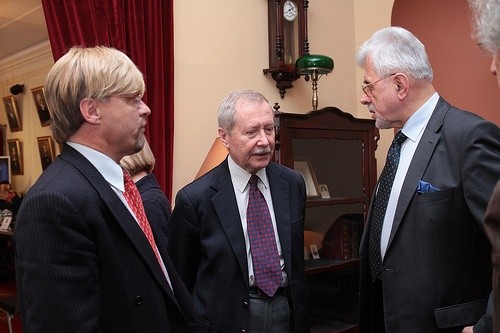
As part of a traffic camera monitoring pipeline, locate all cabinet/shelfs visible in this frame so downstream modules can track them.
[266,103,380,333]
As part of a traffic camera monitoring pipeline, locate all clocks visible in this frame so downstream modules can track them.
[263,0,311,98]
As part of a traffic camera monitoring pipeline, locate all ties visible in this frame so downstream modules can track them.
[246,176,284,297]
[368,132,408,282]
[121,169,162,271]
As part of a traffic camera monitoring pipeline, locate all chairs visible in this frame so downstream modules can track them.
[0,291,18,333]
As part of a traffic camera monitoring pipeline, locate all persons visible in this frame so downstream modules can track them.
[471,0,500,333]
[13,45,199,333]
[167,91,312,333]
[0,135,170,280]
[357,27,500,333]
[321,186,329,197]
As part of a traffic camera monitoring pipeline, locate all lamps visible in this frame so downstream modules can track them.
[294,55,334,112]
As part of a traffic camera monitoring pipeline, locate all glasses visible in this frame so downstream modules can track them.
[360,73,395,97]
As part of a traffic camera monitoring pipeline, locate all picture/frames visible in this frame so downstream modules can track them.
[30,85,53,126]
[37,135,56,172]
[291,158,323,199]
[0,155,11,186]
[3,95,23,133]
[7,138,24,176]
